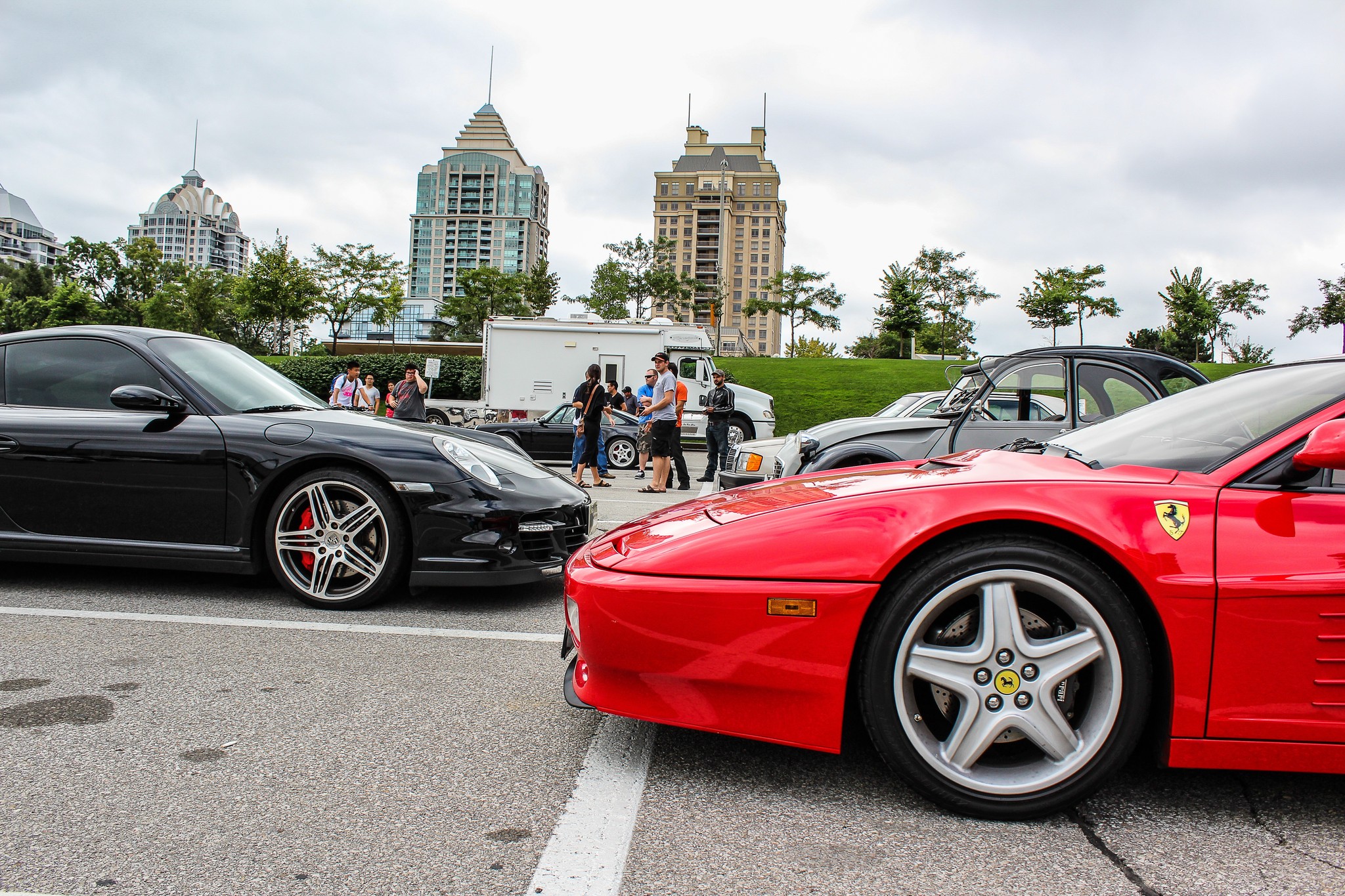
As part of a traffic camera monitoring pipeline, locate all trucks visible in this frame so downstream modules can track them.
[424,315,776,450]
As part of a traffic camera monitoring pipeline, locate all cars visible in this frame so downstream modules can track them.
[559,356,1345,823]
[716,391,1086,491]
[0,324,597,613]
[764,345,1209,486]
[472,402,683,470]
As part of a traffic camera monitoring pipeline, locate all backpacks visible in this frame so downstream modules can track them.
[329,372,358,395]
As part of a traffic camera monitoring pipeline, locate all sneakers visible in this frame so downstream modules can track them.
[599,472,616,479]
[572,472,577,477]
[665,482,673,488]
[634,469,645,479]
[678,482,690,490]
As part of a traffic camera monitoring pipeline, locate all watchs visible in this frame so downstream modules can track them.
[368,404,373,406]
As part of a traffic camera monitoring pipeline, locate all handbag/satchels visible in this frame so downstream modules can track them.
[576,417,584,438]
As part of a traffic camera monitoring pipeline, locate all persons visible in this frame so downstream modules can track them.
[494,409,526,423]
[385,380,396,418]
[329,361,374,411]
[572,371,616,479]
[354,373,380,415]
[696,369,735,482]
[622,386,639,416]
[389,363,428,423]
[607,380,627,412]
[637,352,677,493]
[572,364,613,488]
[666,362,690,490]
[635,369,658,479]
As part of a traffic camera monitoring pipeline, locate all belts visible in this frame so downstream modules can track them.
[709,422,723,426]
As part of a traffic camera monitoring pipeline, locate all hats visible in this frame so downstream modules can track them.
[622,387,631,393]
[651,352,669,361]
[711,369,725,376]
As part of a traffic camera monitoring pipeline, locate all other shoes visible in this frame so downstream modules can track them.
[696,477,714,482]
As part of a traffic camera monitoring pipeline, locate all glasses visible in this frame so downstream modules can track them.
[366,378,374,380]
[406,372,415,375]
[608,386,613,388]
[645,375,657,378]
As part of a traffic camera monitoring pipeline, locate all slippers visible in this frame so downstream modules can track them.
[577,480,592,488]
[660,490,666,493]
[593,480,612,487]
[638,485,660,493]
